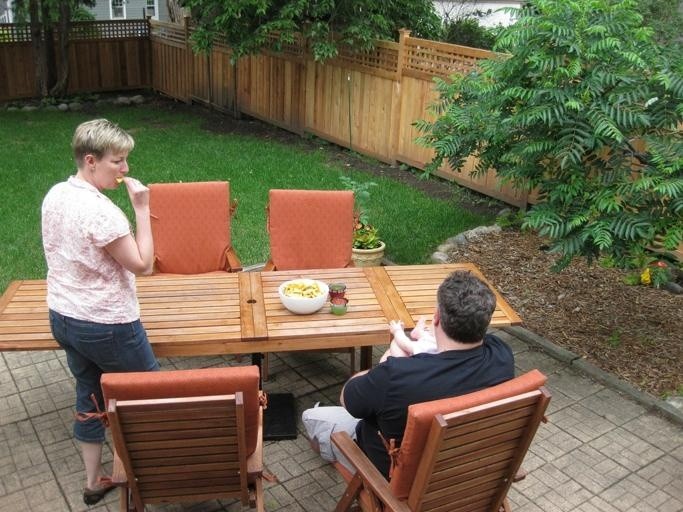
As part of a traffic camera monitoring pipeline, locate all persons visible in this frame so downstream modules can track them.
[41,119,160,503]
[388,317,437,357]
[301,271,514,481]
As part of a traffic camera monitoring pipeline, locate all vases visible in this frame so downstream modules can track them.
[351,239,386,267]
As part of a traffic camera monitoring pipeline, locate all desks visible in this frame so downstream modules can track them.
[0,263,523,444]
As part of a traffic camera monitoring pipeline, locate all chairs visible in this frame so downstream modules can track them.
[328,364,552,512]
[140,179,244,273]
[94,362,267,512]
[262,187,355,388]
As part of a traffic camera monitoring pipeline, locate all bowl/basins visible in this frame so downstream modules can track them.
[279,279,328,316]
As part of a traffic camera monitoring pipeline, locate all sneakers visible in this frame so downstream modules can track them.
[84,477,115,504]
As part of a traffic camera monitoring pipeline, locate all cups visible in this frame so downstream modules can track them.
[327,282,345,297]
[329,296,349,314]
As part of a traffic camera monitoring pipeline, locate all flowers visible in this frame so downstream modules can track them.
[351,204,382,249]
